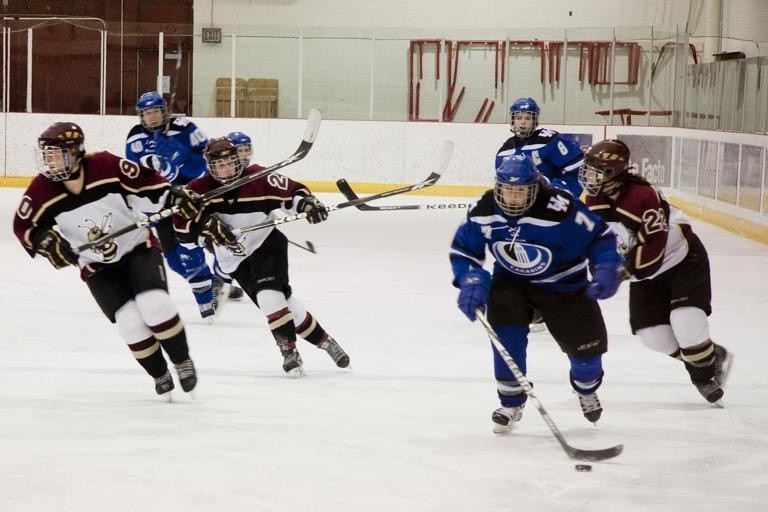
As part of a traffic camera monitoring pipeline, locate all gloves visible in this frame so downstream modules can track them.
[297,196,327,223]
[457,268,492,321]
[588,264,619,299]
[33,229,80,268]
[165,188,206,218]
[201,215,235,245]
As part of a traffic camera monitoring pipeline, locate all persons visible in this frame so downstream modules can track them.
[578,140,728,402]
[13,121,203,395]
[173,137,351,377]
[495,97,584,199]
[217,133,255,302]
[126,92,213,318]
[451,154,620,428]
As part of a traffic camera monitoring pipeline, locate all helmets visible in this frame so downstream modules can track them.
[205,131,252,183]
[495,154,542,217]
[579,139,630,196]
[134,92,168,131]
[36,123,85,183]
[510,98,538,141]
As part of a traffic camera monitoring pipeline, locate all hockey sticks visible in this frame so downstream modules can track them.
[72,108,321,253]
[337,178,476,210]
[475,309,623,459]
[232,141,455,238]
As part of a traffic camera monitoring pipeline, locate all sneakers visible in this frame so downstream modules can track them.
[694,339,727,403]
[578,392,602,422]
[198,274,242,319]
[492,404,524,425]
[324,339,350,368]
[283,345,302,372]
[174,359,196,391]
[154,367,174,394]
